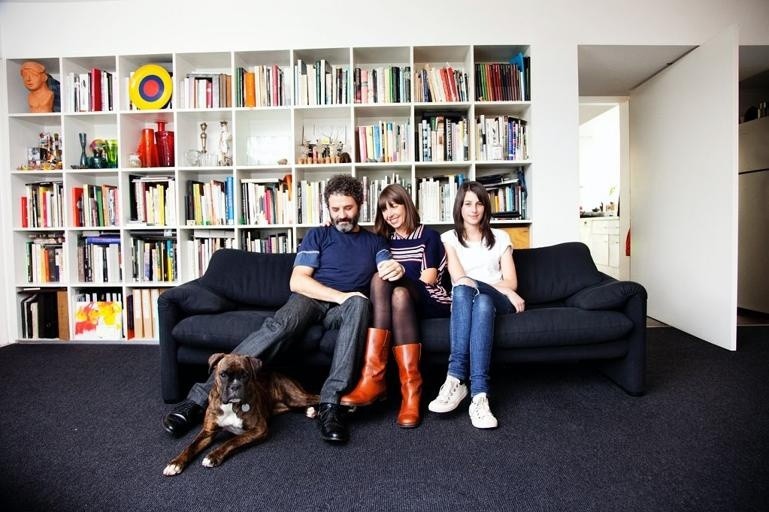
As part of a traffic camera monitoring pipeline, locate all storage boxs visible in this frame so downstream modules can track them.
[71,295,123,340]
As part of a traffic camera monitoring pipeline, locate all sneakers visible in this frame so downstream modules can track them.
[427,375,469,413]
[468,392,498,429]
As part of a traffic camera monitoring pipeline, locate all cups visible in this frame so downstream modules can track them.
[79,133,118,169]
[185,150,218,166]
[139,120,174,167]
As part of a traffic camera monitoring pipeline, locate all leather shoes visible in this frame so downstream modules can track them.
[161,399,205,437]
[315,402,347,442]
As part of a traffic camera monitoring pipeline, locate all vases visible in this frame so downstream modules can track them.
[75,117,173,171]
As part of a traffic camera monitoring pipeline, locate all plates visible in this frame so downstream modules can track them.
[130,64,173,110]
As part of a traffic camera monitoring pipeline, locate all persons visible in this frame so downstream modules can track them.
[428,181,525,429]
[162,175,408,443]
[320,184,453,429]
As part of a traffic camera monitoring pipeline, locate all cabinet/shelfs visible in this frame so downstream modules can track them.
[3,40,534,346]
[580,214,622,282]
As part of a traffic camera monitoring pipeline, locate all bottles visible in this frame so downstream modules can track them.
[39,133,62,170]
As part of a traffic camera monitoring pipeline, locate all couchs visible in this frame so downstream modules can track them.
[154,240,649,411]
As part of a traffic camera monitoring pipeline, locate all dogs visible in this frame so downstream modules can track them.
[162,352,320,479]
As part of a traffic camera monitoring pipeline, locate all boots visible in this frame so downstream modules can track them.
[393,343,424,428]
[340,328,393,406]
